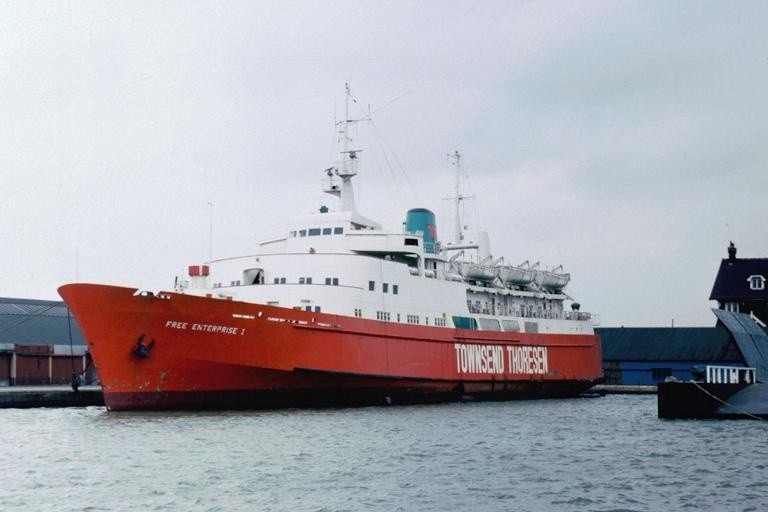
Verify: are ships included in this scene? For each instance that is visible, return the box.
[55,82,606,413]
